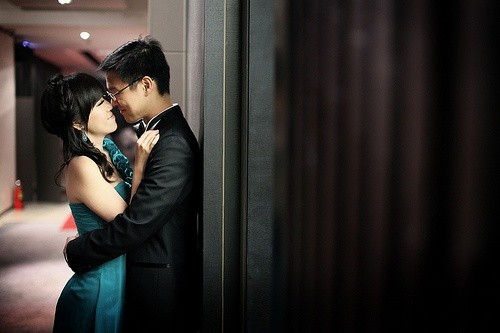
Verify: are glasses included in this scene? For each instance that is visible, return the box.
[105,77,154,102]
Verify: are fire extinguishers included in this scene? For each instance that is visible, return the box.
[13,180,24,210]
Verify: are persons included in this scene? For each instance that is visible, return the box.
[63,35,204,333]
[39,71,159,333]
